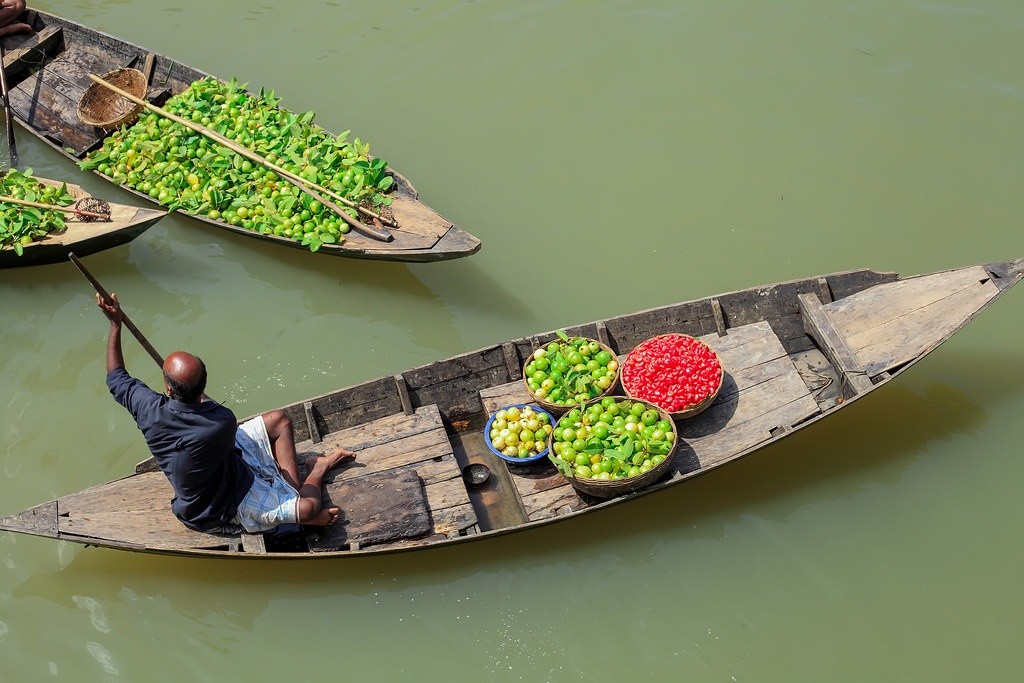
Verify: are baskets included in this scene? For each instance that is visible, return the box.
[484,404,557,465]
[548,395,678,496]
[76,68,148,130]
[620,332,724,420]
[523,337,621,416]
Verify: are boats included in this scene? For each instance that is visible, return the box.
[1,254,1022,561]
[0,167,171,267]
[0,1,483,264]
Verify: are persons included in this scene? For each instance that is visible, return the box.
[95,292,357,534]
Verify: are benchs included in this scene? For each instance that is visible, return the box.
[3,24,62,79]
[798,291,874,395]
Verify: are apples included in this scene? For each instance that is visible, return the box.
[97,83,364,238]
[553,398,674,480]
[525,340,618,406]
[490,406,552,458]
[11,185,57,245]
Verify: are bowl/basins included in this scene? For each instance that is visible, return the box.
[462,463,491,490]
[484,404,557,463]
